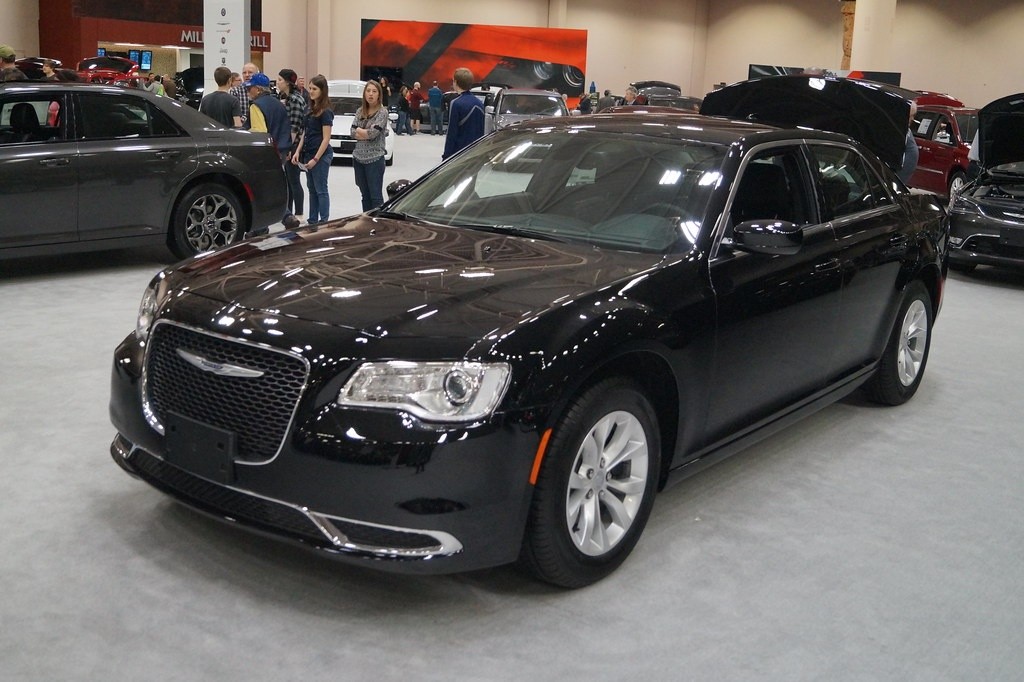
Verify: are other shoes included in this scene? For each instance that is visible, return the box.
[245,227,269,239]
[283,215,300,230]
[294,214,305,223]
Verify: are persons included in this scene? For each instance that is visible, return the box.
[350,79,388,213]
[577,92,593,114]
[803,66,823,74]
[291,74,334,225]
[967,128,980,178]
[595,89,615,114]
[619,86,639,106]
[395,81,424,136]
[0,44,29,81]
[40,60,59,80]
[428,80,447,135]
[379,76,391,108]
[897,99,919,185]
[562,94,568,101]
[199,63,310,239]
[442,67,486,161]
[131,71,177,98]
[481,83,490,91]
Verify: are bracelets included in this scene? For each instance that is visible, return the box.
[313,158,319,163]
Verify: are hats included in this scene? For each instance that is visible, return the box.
[0,46,16,59]
[280,69,297,87]
[245,74,270,86]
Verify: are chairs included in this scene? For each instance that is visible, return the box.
[733,162,798,227]
[502,97,521,113]
[7,103,42,142]
[820,172,850,211]
[100,112,166,135]
[545,175,631,226]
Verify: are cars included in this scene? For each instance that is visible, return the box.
[305,79,400,167]
[485,85,582,134]
[109,74,951,589]
[946,91,1024,285]
[1,82,301,262]
[899,90,982,202]
[574,80,704,114]
[404,86,505,124]
[0,56,137,89]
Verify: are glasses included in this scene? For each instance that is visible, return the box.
[233,80,242,82]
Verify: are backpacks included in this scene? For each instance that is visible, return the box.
[156,84,163,96]
